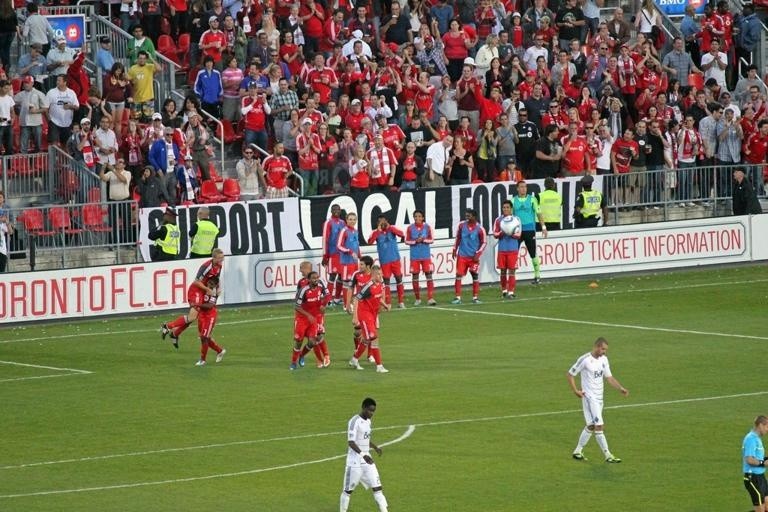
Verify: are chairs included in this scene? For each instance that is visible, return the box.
[236,121,248,137]
[81,203,112,233]
[688,73,705,90]
[217,119,240,143]
[156,34,200,87]
[0,79,61,178]
[200,179,224,202]
[87,188,101,202]
[50,204,80,236]
[198,162,222,181]
[21,207,53,238]
[132,186,141,204]
[221,176,241,201]
[57,169,81,197]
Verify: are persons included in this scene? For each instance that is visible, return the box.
[161,249,226,349]
[568,338,629,463]
[322,180,547,309]
[298,262,331,369]
[346,256,386,363]
[348,267,392,374]
[742,414,768,511]
[2,1,768,274]
[290,271,326,372]
[194,277,227,367]
[340,398,388,512]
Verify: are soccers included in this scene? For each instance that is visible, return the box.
[500,216,521,236]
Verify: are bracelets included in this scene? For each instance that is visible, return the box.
[360,450,368,459]
[760,459,765,467]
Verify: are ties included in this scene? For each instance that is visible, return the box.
[616,114,620,137]
[444,147,446,170]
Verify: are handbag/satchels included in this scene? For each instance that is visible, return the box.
[650,25,660,39]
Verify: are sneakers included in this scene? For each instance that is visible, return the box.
[349,360,364,369]
[334,300,343,306]
[290,363,296,370]
[367,356,375,362]
[509,295,516,299]
[414,300,422,305]
[451,298,462,304]
[678,202,687,208]
[317,361,323,368]
[502,293,508,298]
[195,360,206,366]
[472,299,481,303]
[605,455,622,462]
[531,278,541,285]
[216,349,226,362]
[160,323,169,340]
[323,358,330,367]
[427,298,437,305]
[572,452,588,460]
[686,201,696,207]
[299,354,304,367]
[168,331,179,348]
[399,304,405,308]
[376,366,389,373]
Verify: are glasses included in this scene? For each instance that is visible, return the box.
[407,104,415,106]
[585,126,594,130]
[270,54,279,58]
[1,118,13,125]
[549,105,559,109]
[599,47,608,50]
[165,133,174,136]
[717,110,723,115]
[244,151,253,155]
[519,114,528,117]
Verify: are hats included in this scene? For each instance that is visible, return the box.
[80,118,91,124]
[203,55,214,66]
[301,117,312,125]
[351,99,361,106]
[360,116,372,128]
[224,55,235,65]
[247,80,258,89]
[525,69,536,77]
[100,36,111,43]
[538,15,550,23]
[669,118,678,128]
[512,12,522,19]
[733,166,746,175]
[55,35,66,45]
[184,155,193,161]
[492,80,503,87]
[377,60,387,68]
[710,37,720,45]
[506,157,517,165]
[352,30,363,38]
[464,56,477,65]
[741,3,754,11]
[402,42,415,49]
[165,206,179,217]
[374,114,385,121]
[619,44,629,49]
[152,112,162,120]
[581,175,593,185]
[372,134,383,139]
[31,42,43,53]
[188,111,198,118]
[210,15,219,23]
[22,76,34,83]
[424,35,432,42]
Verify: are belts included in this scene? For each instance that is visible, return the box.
[401,179,415,182]
